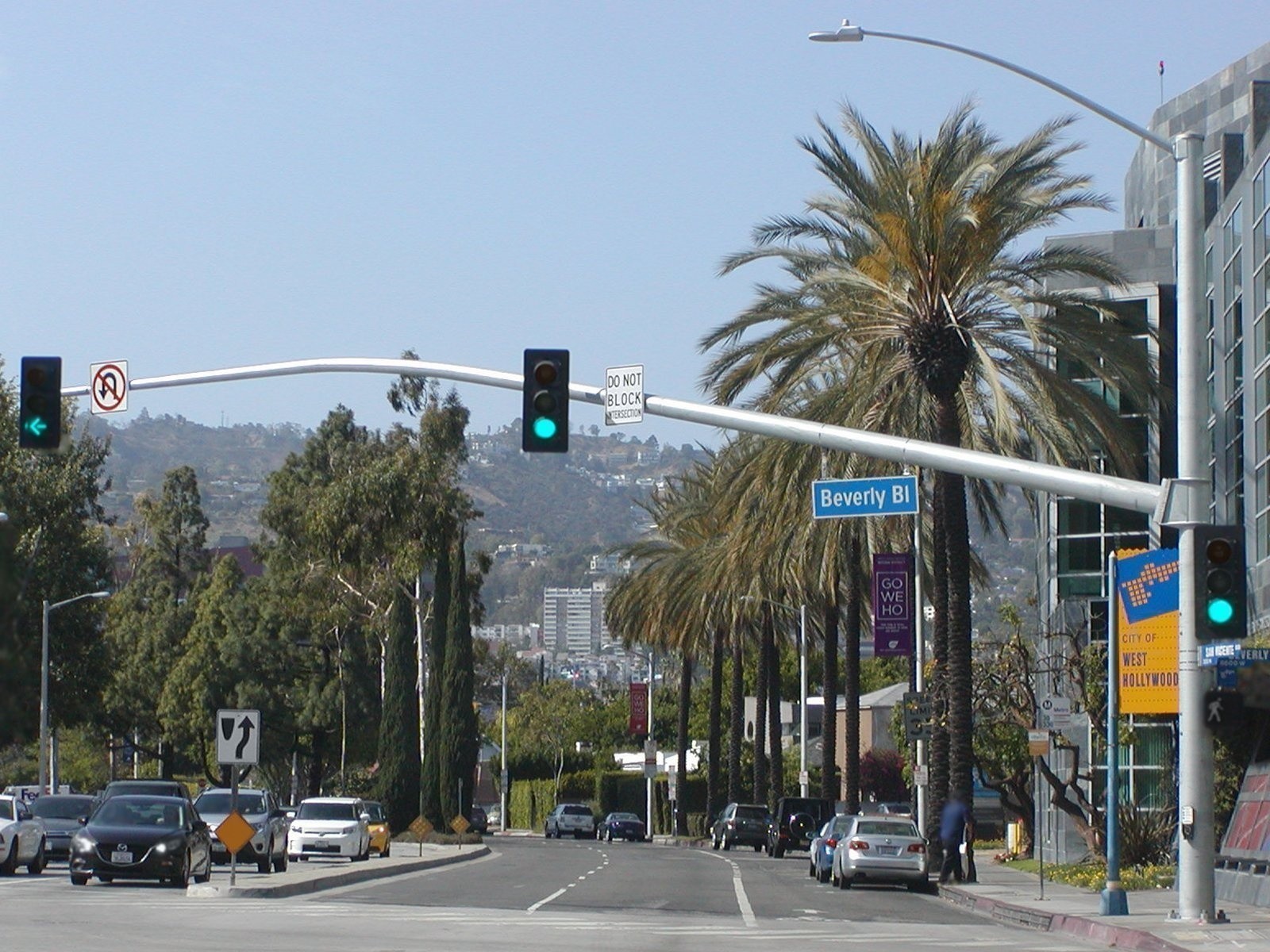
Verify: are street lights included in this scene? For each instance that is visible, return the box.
[38,590,112,799]
[739,595,812,794]
[809,21,1227,925]
[598,641,658,837]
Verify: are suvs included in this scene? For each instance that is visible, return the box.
[286,797,373,862]
[192,785,290,872]
[99,780,193,809]
[543,803,595,839]
[764,796,832,858]
[711,801,771,852]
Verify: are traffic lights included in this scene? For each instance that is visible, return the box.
[19,355,62,452]
[523,348,570,452]
[1194,524,1247,643]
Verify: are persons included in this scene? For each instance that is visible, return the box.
[938,789,977,886]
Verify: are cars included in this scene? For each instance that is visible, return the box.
[597,812,645,842]
[874,802,915,819]
[362,799,392,858]
[68,795,213,888]
[27,795,94,860]
[807,815,931,891]
[0,794,48,877]
[486,804,500,826]
[471,804,490,832]
